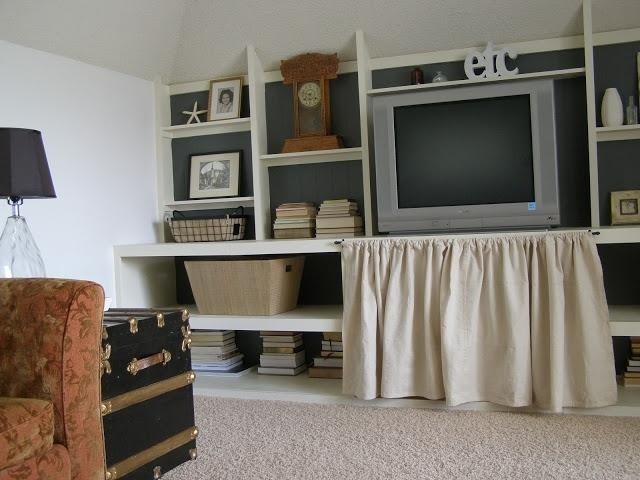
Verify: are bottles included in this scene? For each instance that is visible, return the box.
[410,68,424,85]
[432,71,447,83]
[601,88,624,127]
[626,95,637,125]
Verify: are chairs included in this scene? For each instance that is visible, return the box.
[0,279,106,478]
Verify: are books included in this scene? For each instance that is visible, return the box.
[191,330,342,379]
[617,336,640,387]
[272,198,364,238]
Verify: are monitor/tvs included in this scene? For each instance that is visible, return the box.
[371,77,561,236]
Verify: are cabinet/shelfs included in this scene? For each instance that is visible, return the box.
[148,34,369,239]
[586,29,640,228]
[116,236,640,416]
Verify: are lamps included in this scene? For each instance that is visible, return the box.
[0,127,57,277]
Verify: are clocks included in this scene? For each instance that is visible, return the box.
[279,51,340,153]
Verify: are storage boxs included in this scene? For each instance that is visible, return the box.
[101,307,198,480]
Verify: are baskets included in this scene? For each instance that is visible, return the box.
[166,206,250,243]
[183,255,306,316]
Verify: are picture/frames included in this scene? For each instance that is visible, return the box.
[187,149,242,200]
[206,77,243,121]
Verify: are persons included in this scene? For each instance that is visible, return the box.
[216,88,233,114]
